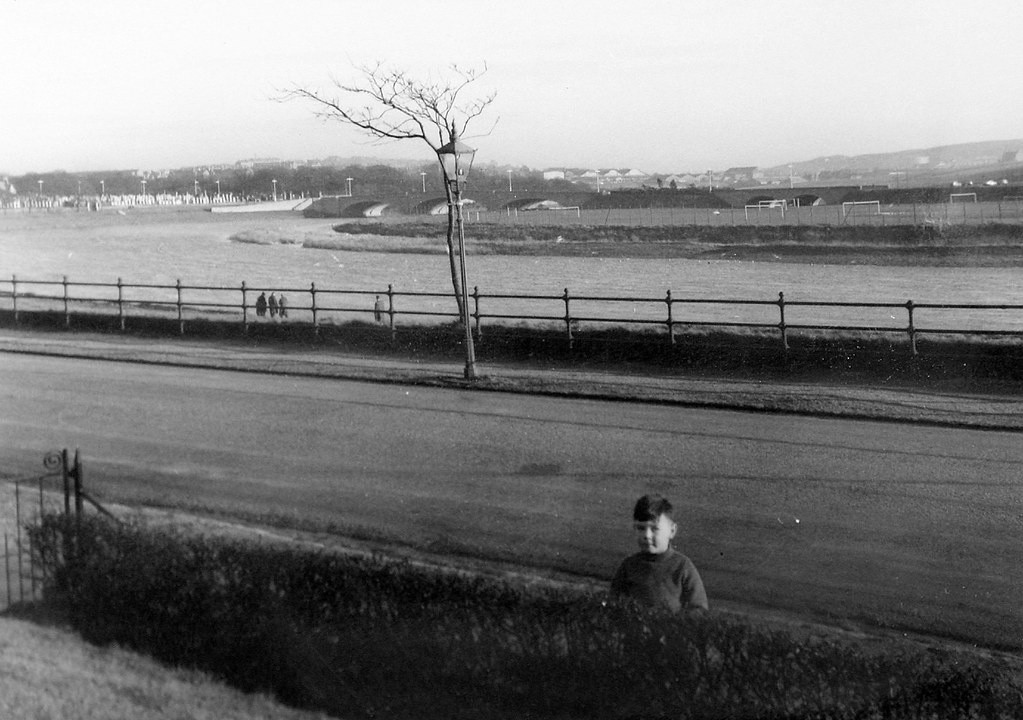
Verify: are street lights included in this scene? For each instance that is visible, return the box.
[33,165,517,194]
[435,120,482,379]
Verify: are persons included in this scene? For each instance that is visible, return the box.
[374,296,384,322]
[255,291,267,316]
[278,293,288,318]
[607,492,708,610]
[268,292,278,318]
